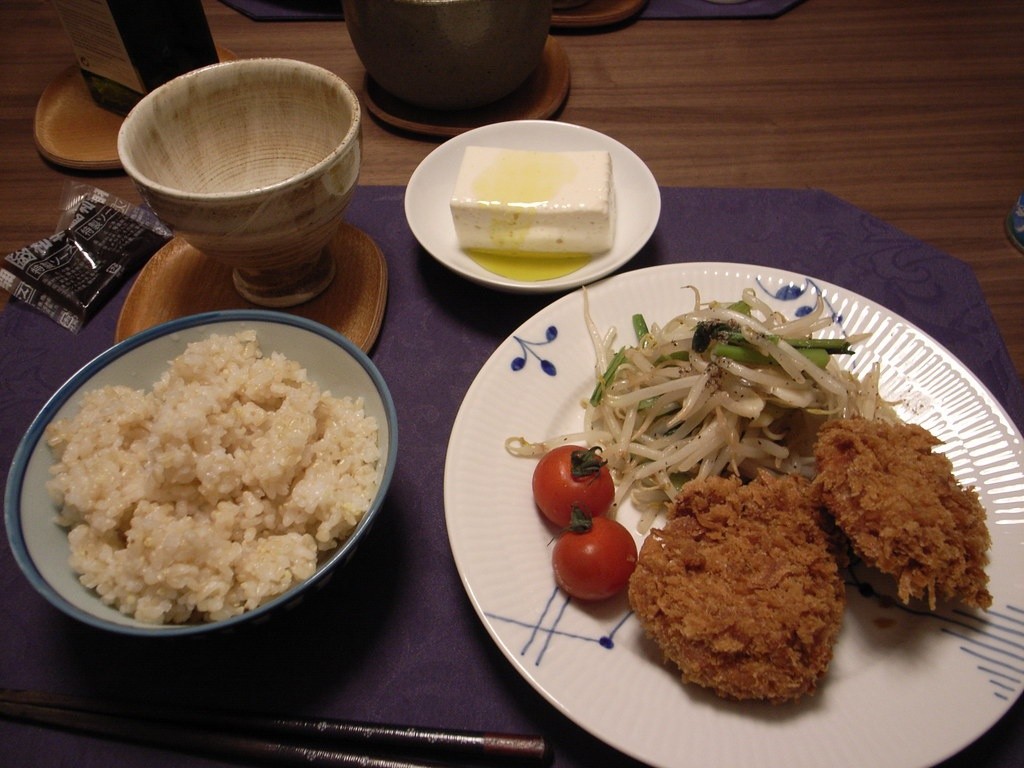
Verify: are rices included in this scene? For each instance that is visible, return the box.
[45,328,381,624]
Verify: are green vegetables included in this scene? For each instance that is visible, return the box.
[726,334,858,356]
[713,347,833,367]
[591,345,625,406]
[726,300,752,316]
[633,313,649,345]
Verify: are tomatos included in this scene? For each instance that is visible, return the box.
[532,445,638,601]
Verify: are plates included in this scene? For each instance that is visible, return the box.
[405,120,662,296]
[112,218,388,368]
[549,0,648,28]
[441,261,1024,768]
[364,32,578,139]
[31,40,242,170]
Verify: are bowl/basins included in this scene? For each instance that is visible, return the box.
[117,53,363,307]
[342,0,548,110]
[4,306,399,639]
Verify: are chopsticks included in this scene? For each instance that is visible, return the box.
[0,682,555,768]
